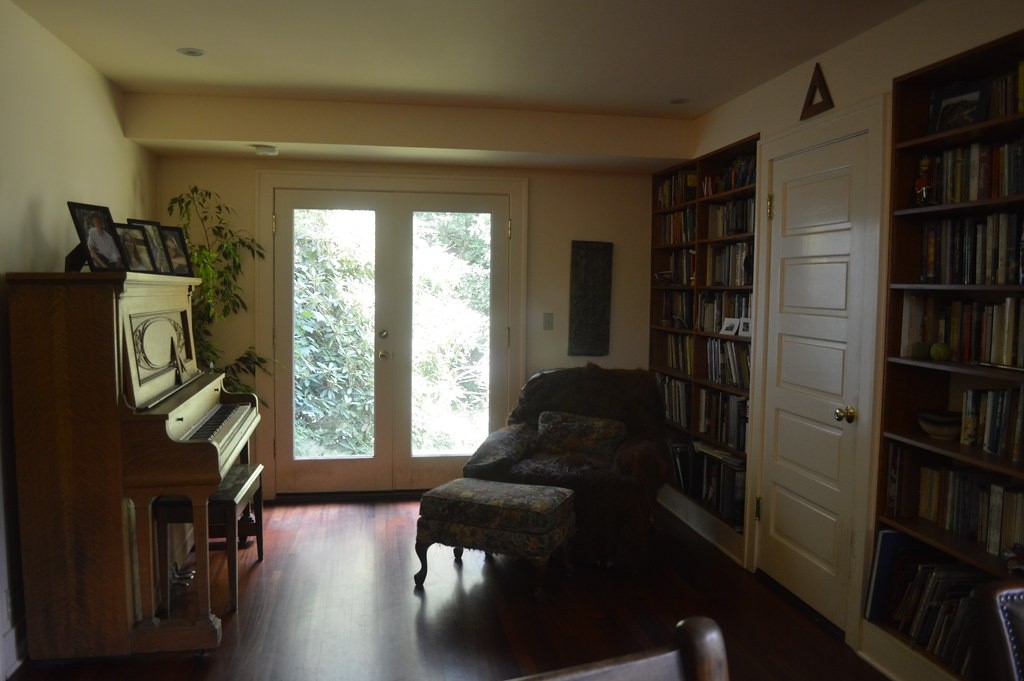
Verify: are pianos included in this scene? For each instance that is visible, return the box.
[1,269,264,663]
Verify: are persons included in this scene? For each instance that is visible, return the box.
[145,229,161,272]
[164,234,188,274]
[87,212,122,268]
[120,232,151,270]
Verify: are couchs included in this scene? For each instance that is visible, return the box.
[464,366,661,571]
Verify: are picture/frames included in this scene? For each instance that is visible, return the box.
[114,223,158,274]
[126,218,174,276]
[161,225,194,278]
[65,201,130,272]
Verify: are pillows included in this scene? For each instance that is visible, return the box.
[535,408,630,459]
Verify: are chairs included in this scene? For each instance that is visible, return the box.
[504,617,730,681]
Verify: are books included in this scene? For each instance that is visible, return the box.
[865,62,1024,681]
[655,155,756,533]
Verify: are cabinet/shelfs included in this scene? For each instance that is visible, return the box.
[647,134,761,567]
[861,29,1024,681]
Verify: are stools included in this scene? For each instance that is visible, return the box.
[152,462,266,613]
[414,478,578,601]
